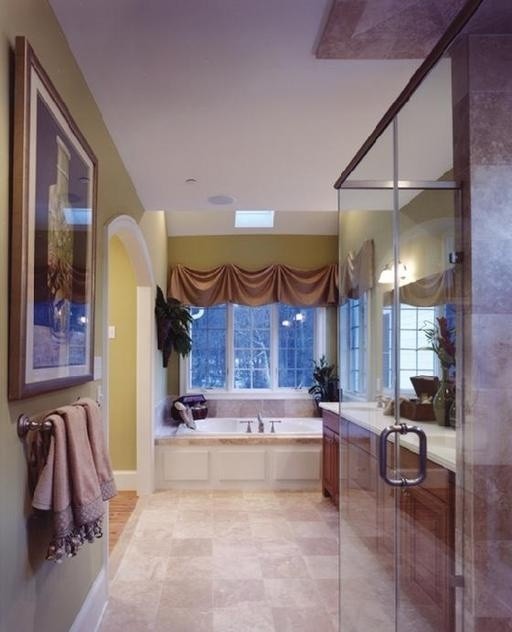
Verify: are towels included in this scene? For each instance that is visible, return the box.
[28,398,120,563]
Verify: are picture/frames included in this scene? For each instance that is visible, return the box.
[7,30,100,406]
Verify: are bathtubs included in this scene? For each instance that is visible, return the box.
[176,417,324,437]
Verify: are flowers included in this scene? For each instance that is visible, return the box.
[417,315,463,370]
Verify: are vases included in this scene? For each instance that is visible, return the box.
[431,367,457,426]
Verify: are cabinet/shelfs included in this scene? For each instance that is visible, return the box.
[338,420,377,547]
[379,440,455,631]
[318,410,347,515]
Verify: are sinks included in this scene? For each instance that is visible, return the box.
[417,433,457,449]
[341,406,377,412]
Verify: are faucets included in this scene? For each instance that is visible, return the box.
[377,396,391,407]
[257,414,264,433]
